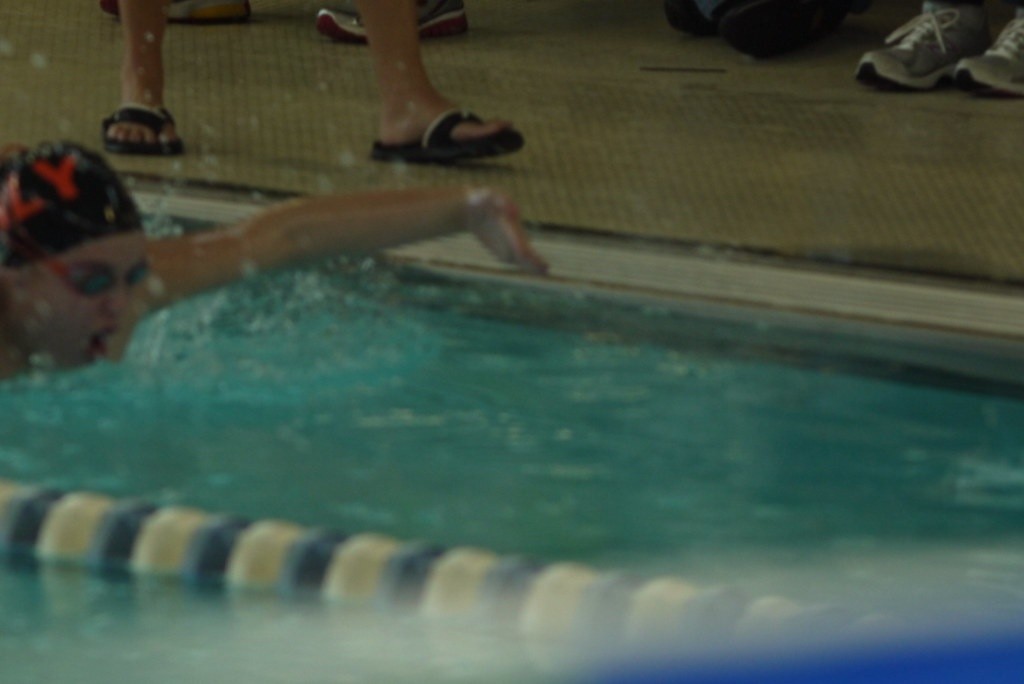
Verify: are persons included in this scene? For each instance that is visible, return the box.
[0,141,549,379]
[100,0,468,44]
[661,0,1024,99]
[101,0,524,161]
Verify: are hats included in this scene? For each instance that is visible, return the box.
[1,141,142,263]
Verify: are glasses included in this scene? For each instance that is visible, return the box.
[17,259,150,293]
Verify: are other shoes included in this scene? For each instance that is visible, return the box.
[318,9,369,47]
[663,1,719,42]
[714,1,854,56]
[419,8,469,38]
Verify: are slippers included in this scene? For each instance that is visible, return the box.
[368,104,522,163]
[102,104,186,159]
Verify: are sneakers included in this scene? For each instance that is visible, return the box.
[856,2,977,93]
[957,14,1024,103]
